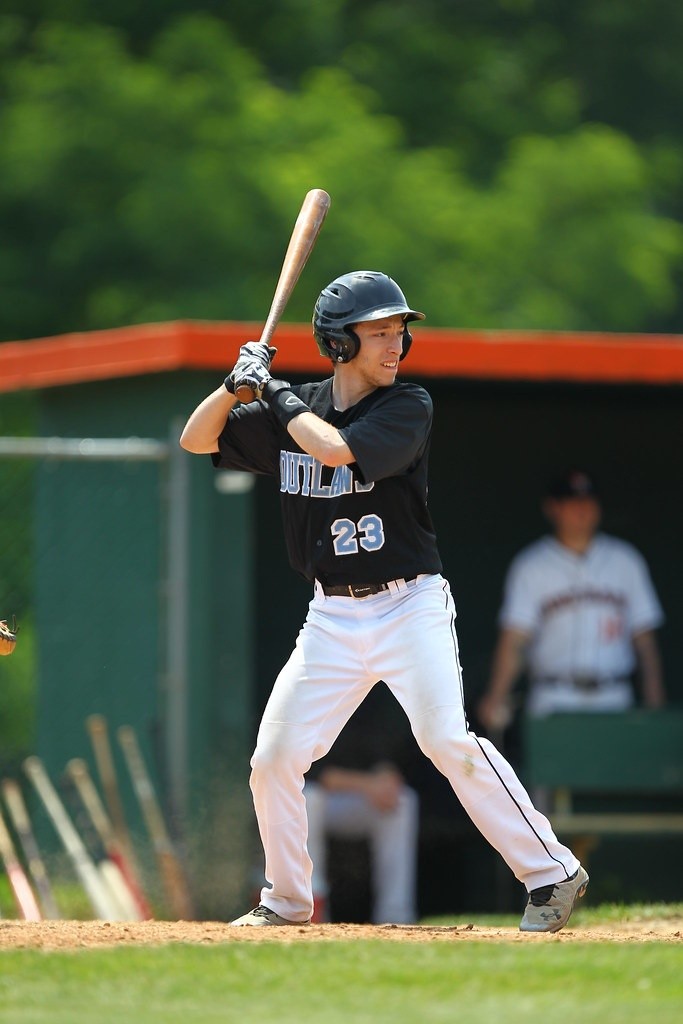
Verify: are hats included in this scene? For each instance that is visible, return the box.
[548,469,602,500]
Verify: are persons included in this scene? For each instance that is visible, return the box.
[276,471,662,926]
[181,271,589,933]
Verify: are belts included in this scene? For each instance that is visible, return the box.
[322,572,417,599]
[538,676,625,689]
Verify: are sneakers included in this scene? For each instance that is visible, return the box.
[519,865,589,933]
[230,907,312,927]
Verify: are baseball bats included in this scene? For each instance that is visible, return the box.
[235,187,331,405]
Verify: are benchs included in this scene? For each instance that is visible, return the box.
[531,714,681,838]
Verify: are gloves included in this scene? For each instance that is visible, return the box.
[223,340,277,395]
[251,362,312,431]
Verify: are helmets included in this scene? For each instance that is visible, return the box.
[312,272,426,364]
